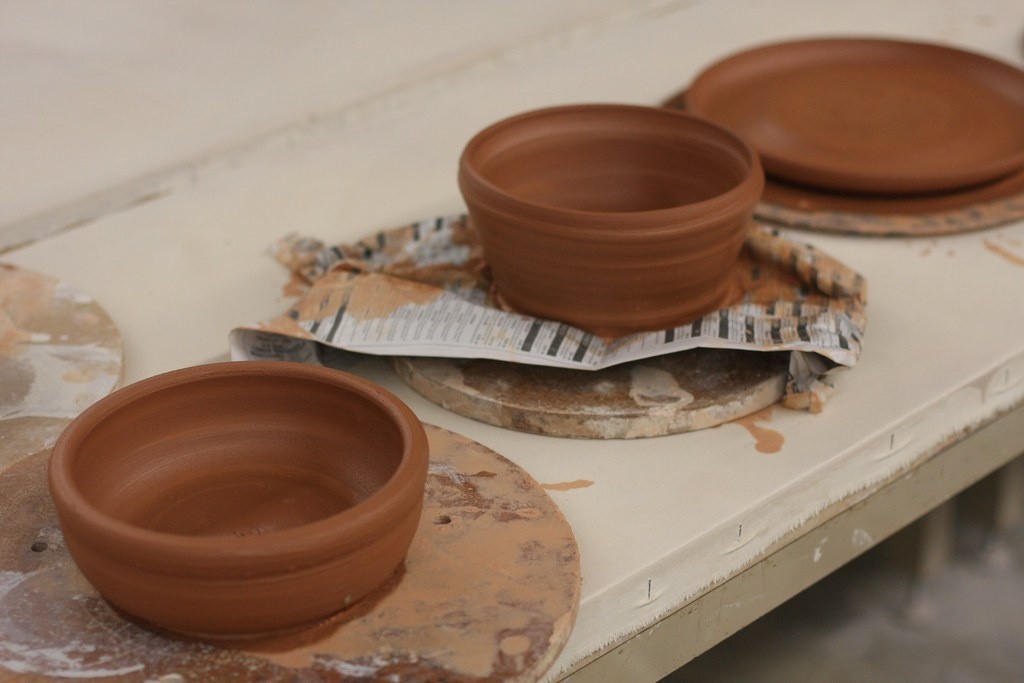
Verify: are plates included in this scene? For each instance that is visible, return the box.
[683,37,1024,199]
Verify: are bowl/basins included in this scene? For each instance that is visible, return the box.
[47,360,429,641]
[457,106,765,338]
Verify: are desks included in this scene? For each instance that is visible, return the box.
[0,0,1024,683]
